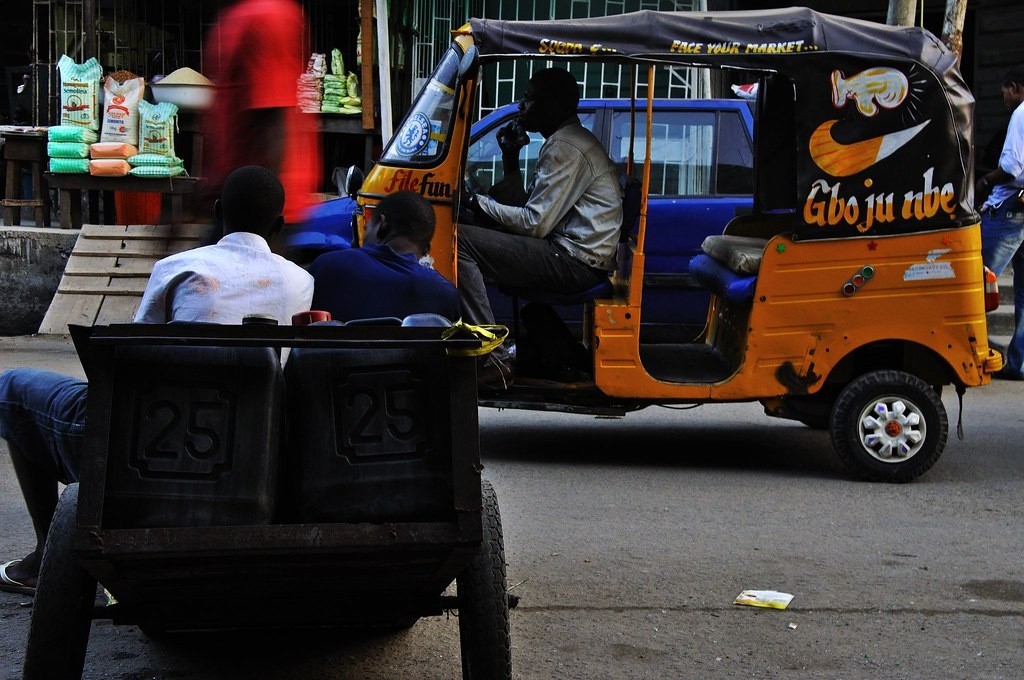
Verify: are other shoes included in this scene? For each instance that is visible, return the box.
[478,360,515,393]
[991,366,1024,380]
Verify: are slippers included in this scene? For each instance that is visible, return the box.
[0,558,38,596]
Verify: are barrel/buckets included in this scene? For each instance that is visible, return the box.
[284,311,441,523]
[114,191,161,225]
[104,315,283,526]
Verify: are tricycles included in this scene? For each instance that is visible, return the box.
[23,313,523,680]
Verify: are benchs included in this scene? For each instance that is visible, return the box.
[687,234,771,299]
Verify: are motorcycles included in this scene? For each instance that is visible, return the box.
[346,7,1005,484]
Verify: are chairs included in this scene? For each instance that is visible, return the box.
[499,178,644,297]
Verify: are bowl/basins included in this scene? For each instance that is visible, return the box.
[151,83,215,109]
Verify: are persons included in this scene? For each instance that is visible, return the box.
[0,166,315,597]
[975,65,1024,380]
[309,191,459,323]
[459,68,623,388]
[204,1,311,175]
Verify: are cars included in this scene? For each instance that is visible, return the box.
[301,96,758,321]
